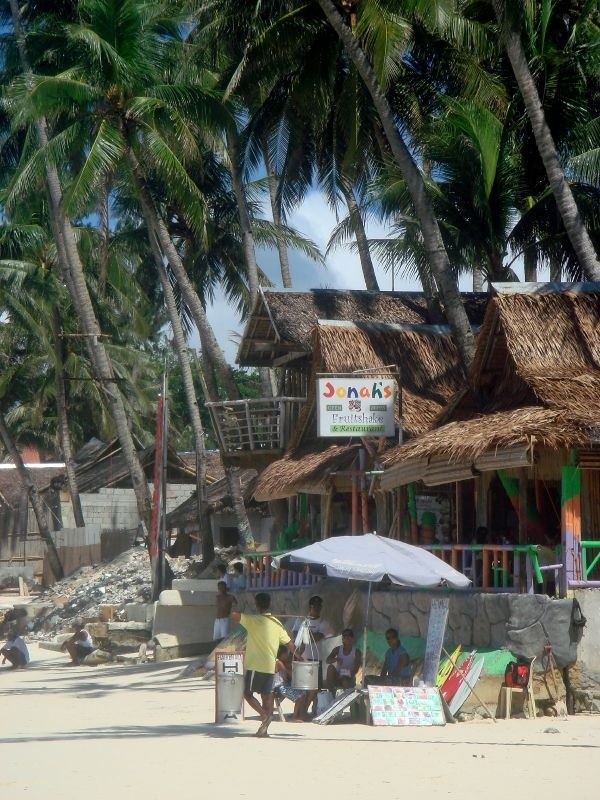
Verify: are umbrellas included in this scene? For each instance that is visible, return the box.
[270,531,472,691]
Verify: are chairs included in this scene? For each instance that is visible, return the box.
[500,655,537,720]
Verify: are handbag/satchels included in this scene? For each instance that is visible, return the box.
[505,659,528,695]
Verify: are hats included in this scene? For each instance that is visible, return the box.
[342,629,354,637]
[310,596,322,605]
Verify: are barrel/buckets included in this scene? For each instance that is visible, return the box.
[292,626,320,691]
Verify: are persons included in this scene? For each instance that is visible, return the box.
[0,630,29,670]
[182,521,203,559]
[359,628,412,687]
[61,617,94,667]
[418,524,559,596]
[214,562,362,738]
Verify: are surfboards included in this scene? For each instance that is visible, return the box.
[438,645,484,716]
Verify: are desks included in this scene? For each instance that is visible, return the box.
[358,688,372,724]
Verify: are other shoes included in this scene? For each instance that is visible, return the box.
[256,715,273,737]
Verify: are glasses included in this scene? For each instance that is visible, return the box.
[386,636,395,640]
[311,605,321,609]
